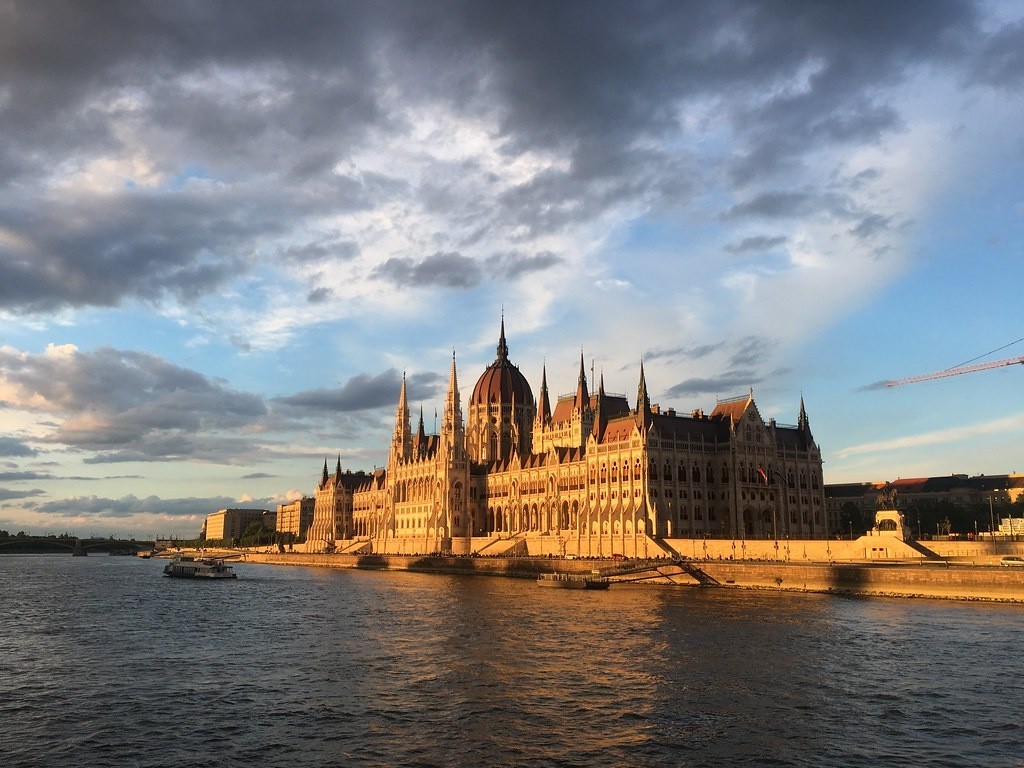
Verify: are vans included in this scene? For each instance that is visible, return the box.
[194,554,203,561]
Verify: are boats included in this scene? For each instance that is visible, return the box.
[162,556,237,579]
[536,572,611,589]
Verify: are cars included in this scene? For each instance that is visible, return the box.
[1000,556,1024,567]
[610,554,624,559]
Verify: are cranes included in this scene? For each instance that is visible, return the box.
[885,338,1024,388]
